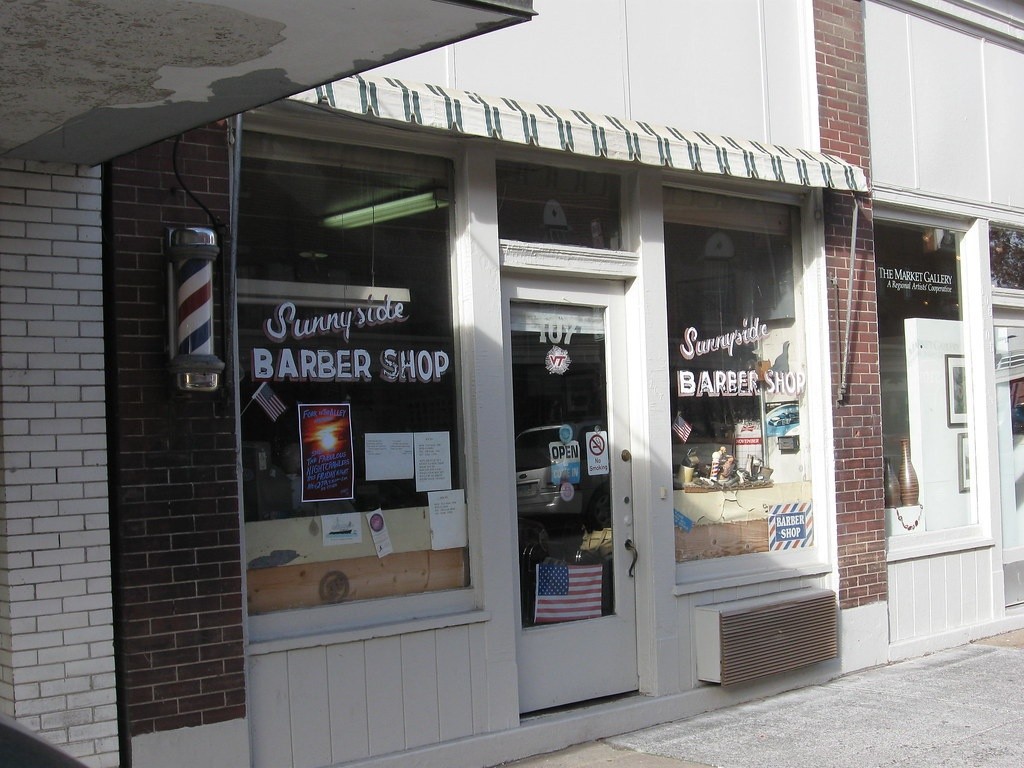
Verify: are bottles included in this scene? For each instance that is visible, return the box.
[884,456,900,508]
[898,439,919,506]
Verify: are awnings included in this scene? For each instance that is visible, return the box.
[289,76,871,194]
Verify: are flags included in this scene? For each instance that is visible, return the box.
[533,563,603,622]
[672,417,692,443]
[251,382,286,422]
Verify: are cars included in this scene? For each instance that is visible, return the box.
[766,402,802,456]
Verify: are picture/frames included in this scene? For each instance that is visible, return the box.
[958,433,971,494]
[946,354,968,429]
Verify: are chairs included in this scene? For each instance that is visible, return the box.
[242,441,306,520]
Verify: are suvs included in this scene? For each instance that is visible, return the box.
[514,420,614,535]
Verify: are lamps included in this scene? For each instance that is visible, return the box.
[160,226,224,393]
[317,187,450,232]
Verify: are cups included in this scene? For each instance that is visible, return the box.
[677,465,694,484]
